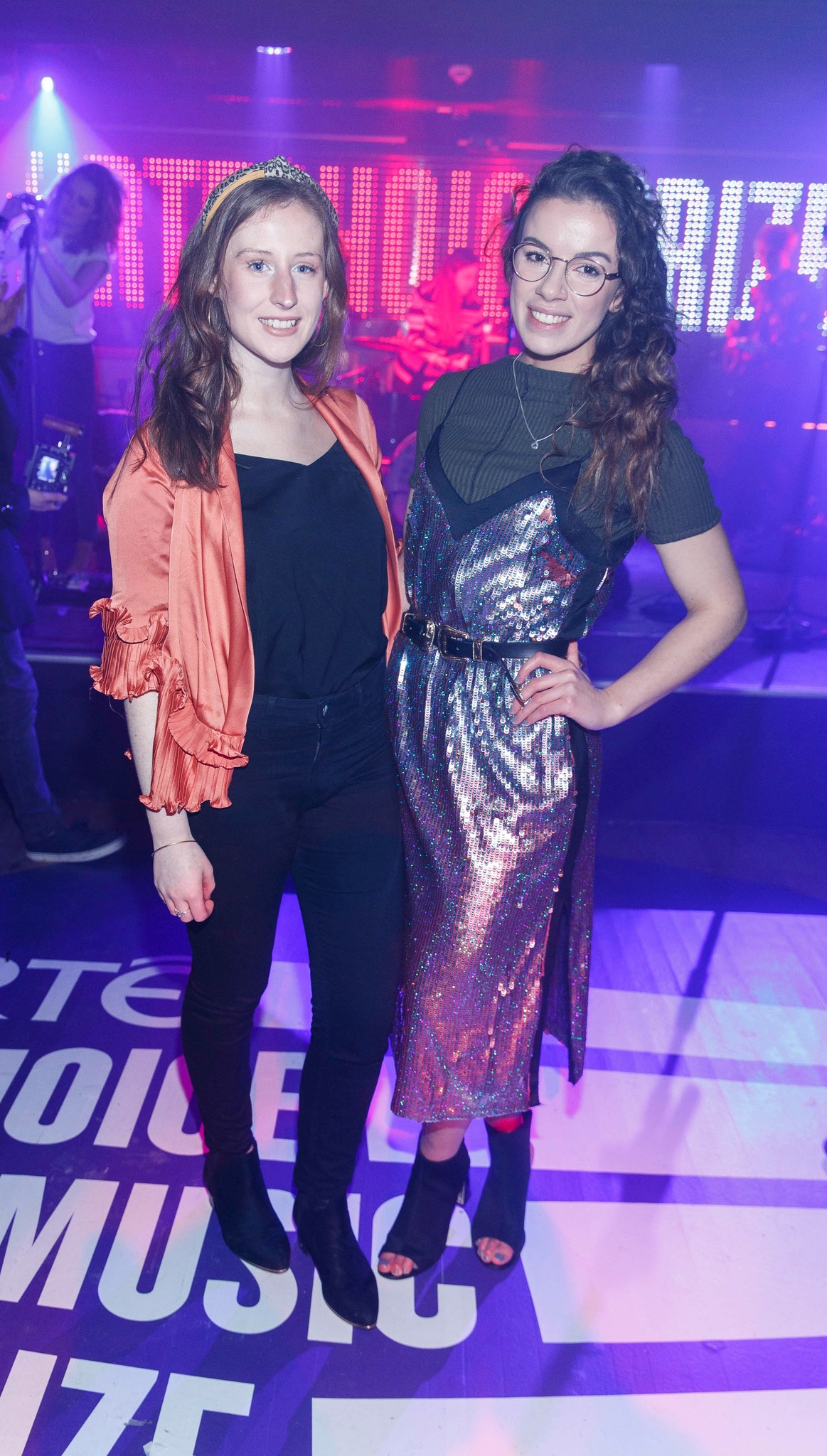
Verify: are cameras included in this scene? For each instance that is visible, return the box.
[26,445,76,494]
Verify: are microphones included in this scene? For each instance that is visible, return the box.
[20,193,49,209]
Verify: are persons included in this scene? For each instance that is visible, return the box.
[0,161,123,868]
[724,227,817,567]
[389,250,489,431]
[379,149,750,1280]
[91,165,406,1330]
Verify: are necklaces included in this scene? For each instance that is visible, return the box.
[512,352,590,448]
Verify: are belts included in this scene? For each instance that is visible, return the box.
[400,613,566,662]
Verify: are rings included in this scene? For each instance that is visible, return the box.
[174,908,190,917]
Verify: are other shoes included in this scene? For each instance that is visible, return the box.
[24,820,126,863]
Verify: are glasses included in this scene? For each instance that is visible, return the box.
[511,243,623,296]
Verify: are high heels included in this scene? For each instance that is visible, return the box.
[293,1191,378,1328]
[204,1138,292,1274]
[466,1109,532,1268]
[378,1137,472,1281]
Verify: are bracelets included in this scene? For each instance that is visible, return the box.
[38,247,49,256]
[151,840,196,856]
[0,215,9,230]
